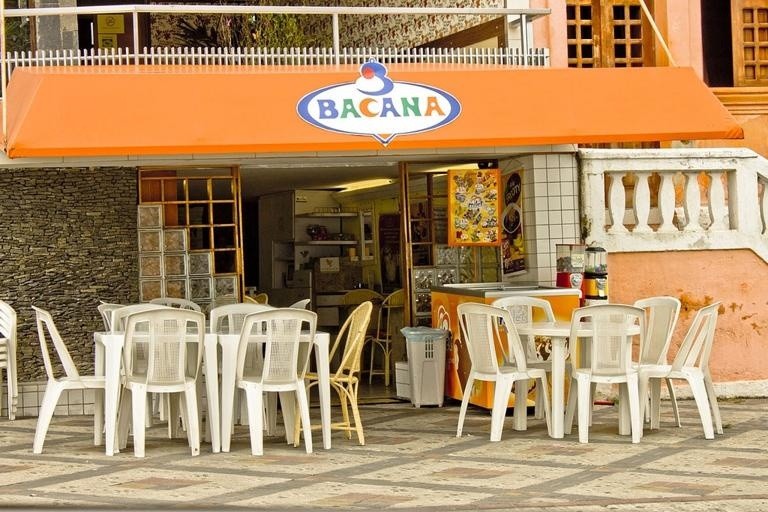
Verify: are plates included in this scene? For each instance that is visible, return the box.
[500,202,522,234]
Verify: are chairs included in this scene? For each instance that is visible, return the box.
[210,303,273,333]
[0,300,20,422]
[149,297,202,315]
[228,306,315,455]
[242,293,269,304]
[289,298,311,309]
[26,304,122,455]
[292,301,375,447]
[456,302,551,443]
[99,303,125,331]
[113,310,207,455]
[490,295,568,421]
[617,296,682,429]
[343,288,404,386]
[567,304,647,445]
[639,301,724,440]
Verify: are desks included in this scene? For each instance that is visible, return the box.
[505,320,643,439]
[92,328,333,457]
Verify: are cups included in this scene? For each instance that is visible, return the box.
[347,247,355,257]
[308,224,344,240]
[353,282,365,288]
[365,248,369,256]
[313,207,359,213]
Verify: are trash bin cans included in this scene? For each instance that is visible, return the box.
[399,326,449,408]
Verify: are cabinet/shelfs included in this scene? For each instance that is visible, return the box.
[256,188,361,325]
